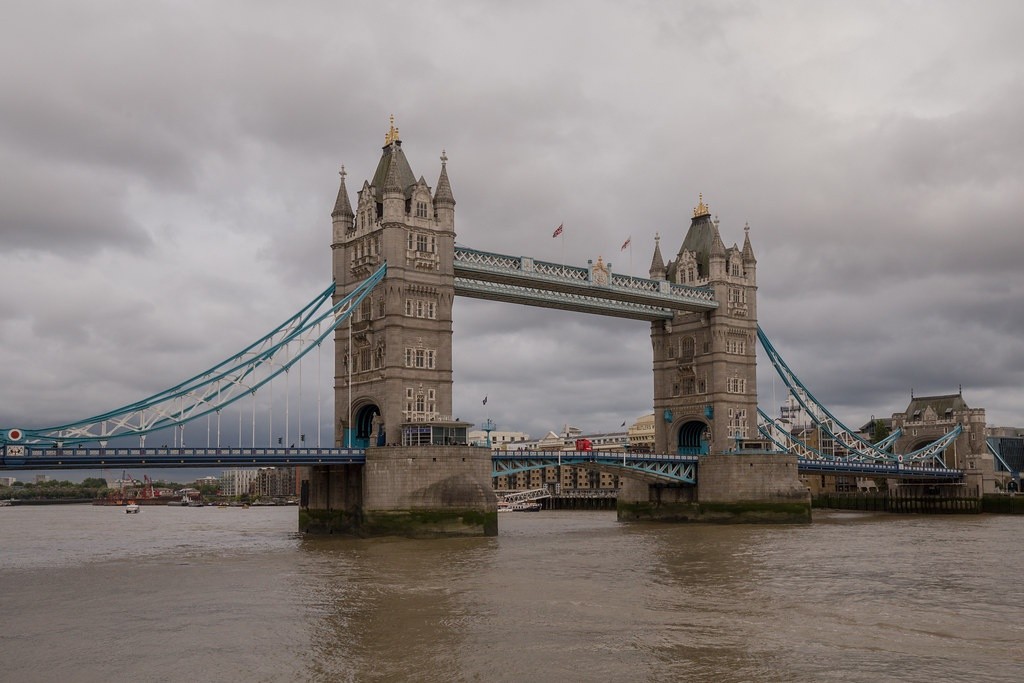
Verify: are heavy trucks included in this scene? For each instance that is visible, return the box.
[575,438,593,451]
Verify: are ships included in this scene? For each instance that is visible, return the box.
[92,470,173,505]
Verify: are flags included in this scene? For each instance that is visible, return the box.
[621,422,625,427]
[621,236,631,252]
[482,395,487,405]
[553,223,563,238]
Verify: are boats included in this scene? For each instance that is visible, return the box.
[125,502,140,514]
[166,496,298,509]
[498,498,542,513]
[0,499,21,507]
